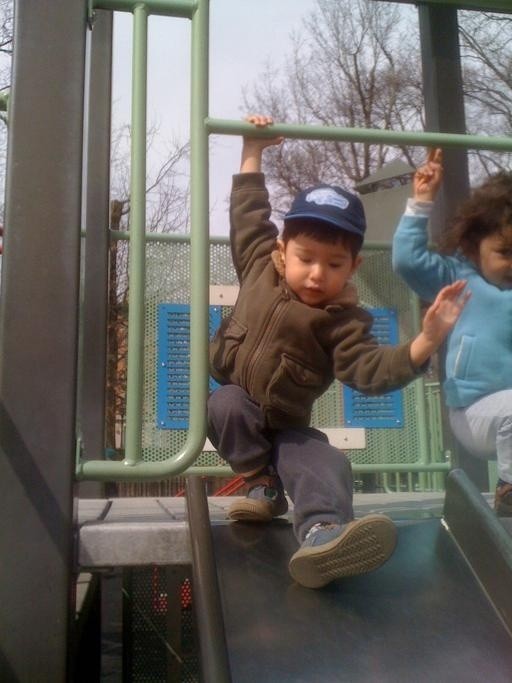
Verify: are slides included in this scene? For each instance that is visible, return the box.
[185,466,510,681]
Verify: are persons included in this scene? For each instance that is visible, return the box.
[204,113,474,592]
[388,146,511,518]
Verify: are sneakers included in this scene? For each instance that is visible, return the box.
[230,484,290,521]
[288,514,399,591]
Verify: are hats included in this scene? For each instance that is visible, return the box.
[280,183,367,239]
